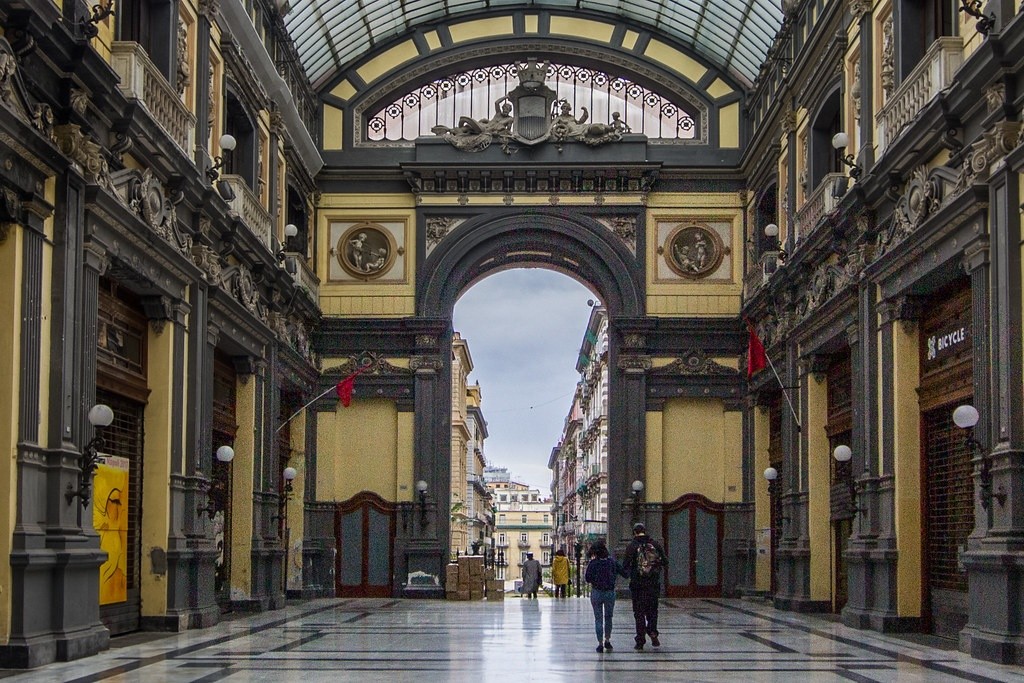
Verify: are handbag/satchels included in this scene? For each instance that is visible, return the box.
[537,572,542,585]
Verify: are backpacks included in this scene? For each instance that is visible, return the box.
[636,541,661,577]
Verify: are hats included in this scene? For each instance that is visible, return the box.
[526,552,533,557]
[632,523,646,536]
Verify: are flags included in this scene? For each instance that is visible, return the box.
[746,318,767,376]
[339,364,368,409]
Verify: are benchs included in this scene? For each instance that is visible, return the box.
[402,587,443,598]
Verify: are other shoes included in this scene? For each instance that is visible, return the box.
[605,641,613,649]
[596,645,604,653]
[650,631,660,646]
[634,642,644,650]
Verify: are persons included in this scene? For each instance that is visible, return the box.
[364,248,387,273]
[349,232,367,270]
[678,245,698,272]
[586,540,627,655]
[521,551,543,599]
[550,549,569,600]
[609,111,631,140]
[693,231,708,268]
[489,91,513,130]
[544,102,576,139]
[624,522,663,652]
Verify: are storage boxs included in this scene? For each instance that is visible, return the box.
[445,555,505,601]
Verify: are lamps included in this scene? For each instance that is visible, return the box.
[417,480,430,532]
[764,225,788,262]
[64,403,113,510]
[205,135,237,184]
[263,468,296,508]
[834,444,867,516]
[832,133,862,182]
[197,445,234,519]
[764,467,791,524]
[952,404,1007,508]
[633,481,644,517]
[276,224,298,264]
